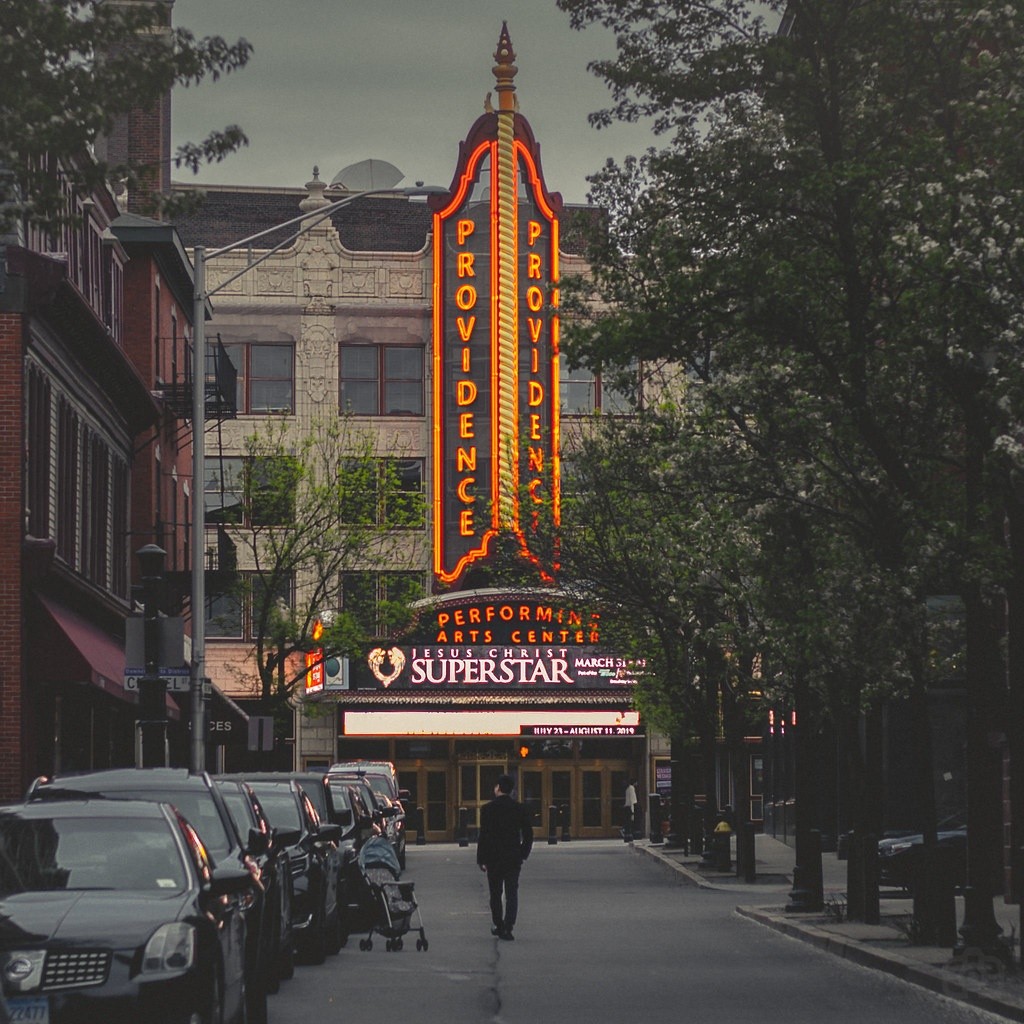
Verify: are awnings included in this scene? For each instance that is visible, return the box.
[25,584,182,724]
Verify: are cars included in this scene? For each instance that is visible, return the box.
[2,798,258,1024]
[878,819,969,895]
[29,758,411,979]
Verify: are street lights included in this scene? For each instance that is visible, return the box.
[192,178,450,782]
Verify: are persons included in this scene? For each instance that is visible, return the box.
[623,778,638,843]
[476,775,534,941]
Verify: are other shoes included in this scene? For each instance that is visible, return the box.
[491,925,514,941]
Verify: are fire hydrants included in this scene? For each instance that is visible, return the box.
[714,821,734,873]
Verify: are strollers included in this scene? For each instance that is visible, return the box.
[347,832,431,955]
